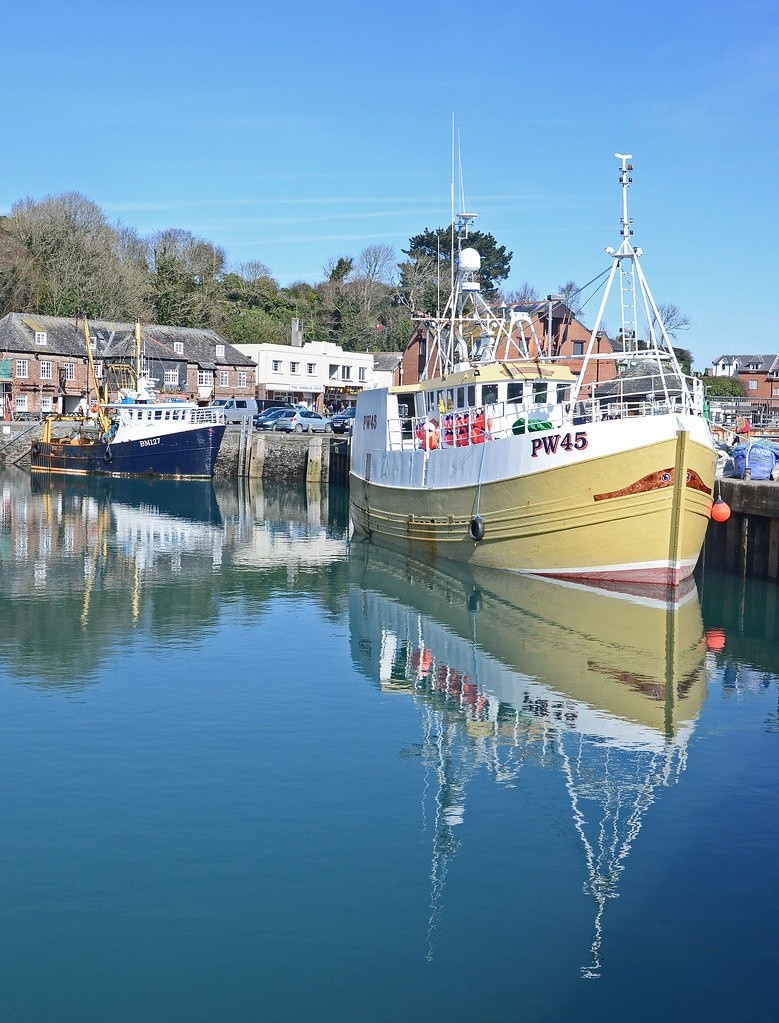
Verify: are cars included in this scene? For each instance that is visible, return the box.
[277,411,333,433]
[254,406,285,427]
[254,408,294,432]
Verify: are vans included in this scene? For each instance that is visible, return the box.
[331,407,356,434]
[207,398,258,424]
[285,403,308,411]
[257,399,295,414]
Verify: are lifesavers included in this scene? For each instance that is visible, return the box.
[33,442,41,453]
[468,519,485,542]
[104,451,112,461]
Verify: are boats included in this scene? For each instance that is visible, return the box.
[347,113,722,586]
[29,471,226,633]
[347,537,712,983]
[29,309,230,479]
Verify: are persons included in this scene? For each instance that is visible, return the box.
[732,412,750,446]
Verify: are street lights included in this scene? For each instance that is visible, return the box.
[595,334,602,391]
[547,294,565,363]
[620,329,633,369]
[728,363,731,377]
